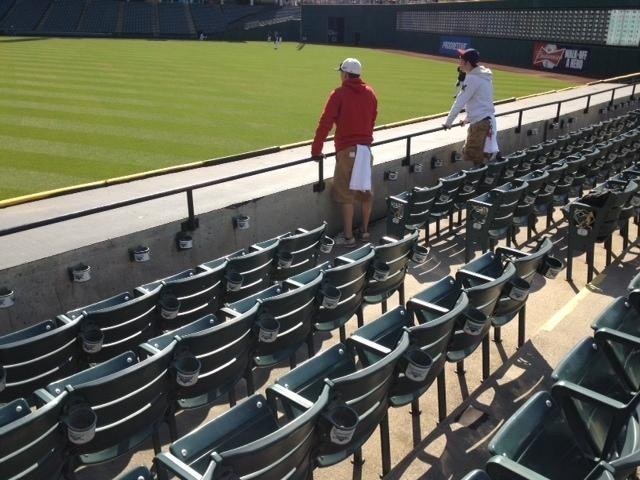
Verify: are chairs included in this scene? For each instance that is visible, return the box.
[386,112,640,283]
[2,1,301,36]
[0,220,640,479]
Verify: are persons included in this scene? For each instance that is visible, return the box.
[453,64,465,98]
[309,55,380,249]
[267,29,272,42]
[440,49,496,169]
[273,30,280,49]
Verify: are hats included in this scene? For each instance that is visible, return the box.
[456,48,481,65]
[334,57,362,75]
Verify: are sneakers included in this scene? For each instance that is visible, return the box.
[332,232,358,248]
[352,224,371,242]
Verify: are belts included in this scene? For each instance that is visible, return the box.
[483,116,491,120]
